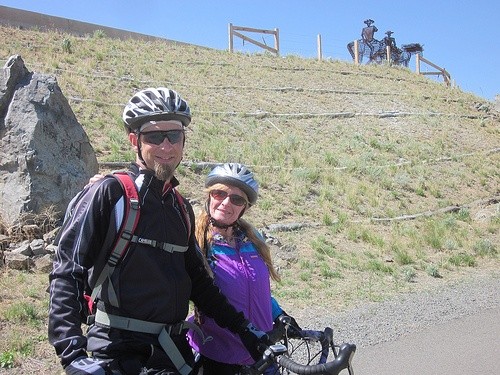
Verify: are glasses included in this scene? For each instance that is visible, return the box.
[210,189,247,206]
[139,129,183,146]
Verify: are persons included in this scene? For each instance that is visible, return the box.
[361,19,378,60]
[186,162,302,375]
[48,87,272,375]
[379,31,395,58]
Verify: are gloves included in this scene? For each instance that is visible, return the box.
[64,355,107,375]
[237,319,275,363]
[279,315,302,340]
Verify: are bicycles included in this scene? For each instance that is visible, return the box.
[241,342,357,375]
[266,314,337,365]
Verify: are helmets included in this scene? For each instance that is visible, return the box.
[204,162,258,207]
[122,87,191,129]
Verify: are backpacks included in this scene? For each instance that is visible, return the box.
[45,170,193,327]
